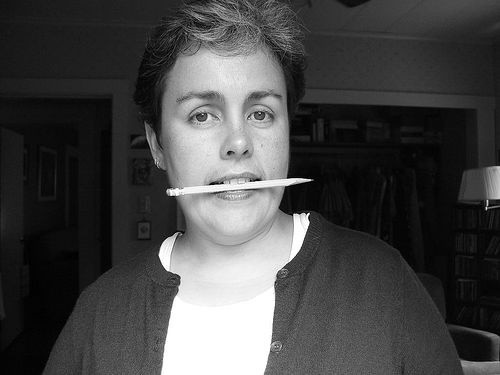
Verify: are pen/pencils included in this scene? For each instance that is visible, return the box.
[165,177,314,196]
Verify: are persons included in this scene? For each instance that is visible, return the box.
[42,0,464,375]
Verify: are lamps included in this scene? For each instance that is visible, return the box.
[457,166,500,211]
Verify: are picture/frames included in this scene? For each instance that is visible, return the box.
[36,145,58,203]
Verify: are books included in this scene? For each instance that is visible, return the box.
[452,231,478,324]
[478,295,500,333]
[291,113,445,147]
[454,203,500,230]
[484,235,500,257]
